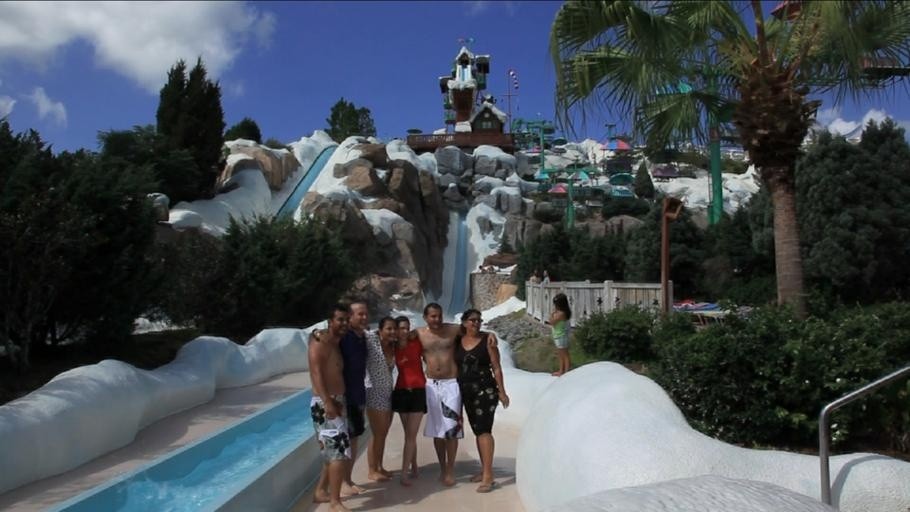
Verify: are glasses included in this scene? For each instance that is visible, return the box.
[469,319,483,323]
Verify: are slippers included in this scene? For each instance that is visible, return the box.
[476,482,498,492]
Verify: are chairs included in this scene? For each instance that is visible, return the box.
[673,299,760,325]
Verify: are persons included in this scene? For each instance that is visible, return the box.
[454,308,510,494]
[479,265,496,274]
[392,316,427,486]
[312,299,371,496]
[361,315,417,481]
[408,302,498,486]
[529,269,542,283]
[544,292,572,376]
[307,303,355,512]
[540,269,551,284]
